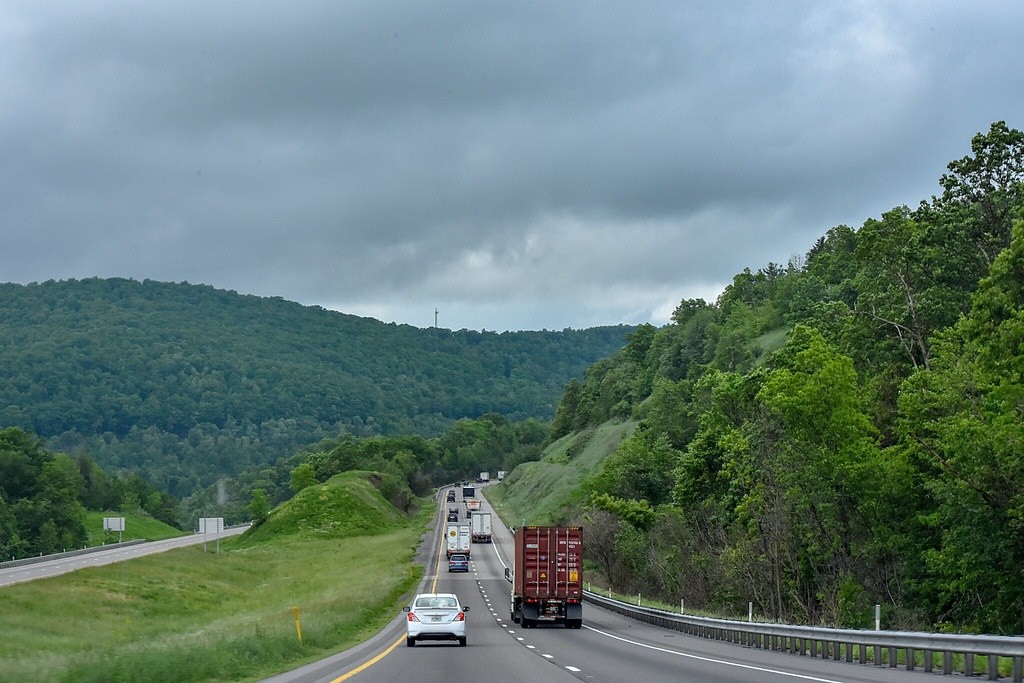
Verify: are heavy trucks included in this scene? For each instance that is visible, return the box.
[504,525,584,629]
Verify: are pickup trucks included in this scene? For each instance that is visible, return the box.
[455,480,470,487]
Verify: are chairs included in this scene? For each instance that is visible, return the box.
[420,600,430,607]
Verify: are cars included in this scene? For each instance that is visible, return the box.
[447,513,458,522]
[446,490,456,502]
[448,554,469,572]
[402,592,470,647]
[449,506,458,514]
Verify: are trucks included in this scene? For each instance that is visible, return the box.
[463,488,474,498]
[463,499,482,518]
[480,472,489,483]
[469,511,492,543]
[444,521,472,559]
[498,471,509,481]
